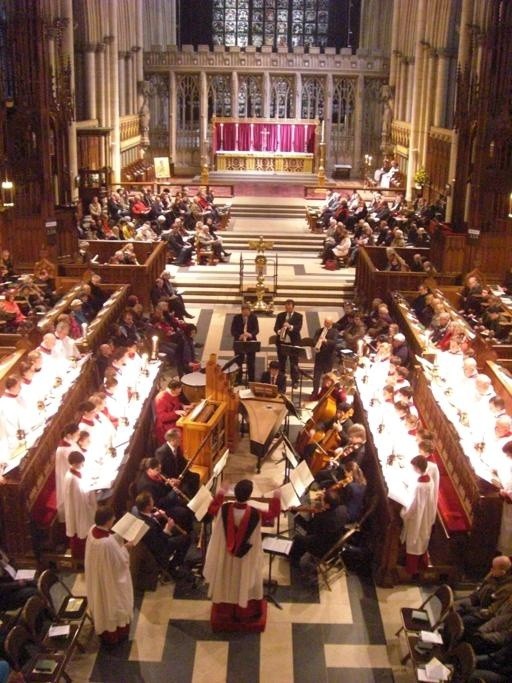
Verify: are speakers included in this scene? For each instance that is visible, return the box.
[170,162,175,179]
[331,164,352,180]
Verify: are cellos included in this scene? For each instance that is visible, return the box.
[295,380,342,459]
[308,407,355,479]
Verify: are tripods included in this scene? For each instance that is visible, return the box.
[269,416,301,538]
[295,373,312,411]
[264,552,284,613]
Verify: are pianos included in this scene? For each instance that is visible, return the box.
[176,398,229,478]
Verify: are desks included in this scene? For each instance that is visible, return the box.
[334,163,351,180]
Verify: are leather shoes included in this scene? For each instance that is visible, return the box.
[190,248,232,264]
[186,313,204,348]
[293,382,318,399]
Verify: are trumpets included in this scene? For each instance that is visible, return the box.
[314,327,329,353]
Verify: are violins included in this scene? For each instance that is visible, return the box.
[281,502,329,514]
[330,477,353,490]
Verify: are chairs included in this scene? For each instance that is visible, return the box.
[394,582,476,682]
[309,488,380,593]
[194,240,214,265]
[334,246,353,267]
[3,567,93,682]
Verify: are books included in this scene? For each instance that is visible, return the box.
[411,610,428,623]
[413,630,443,655]
[417,657,451,682]
[15,569,36,581]
[36,660,55,671]
[50,624,70,639]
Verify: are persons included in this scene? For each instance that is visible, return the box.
[75,188,231,264]
[204,479,264,620]
[315,189,448,263]
[84,506,133,646]
[1,552,37,605]
[0,243,512,574]
[378,158,391,185]
[380,160,400,188]
[452,555,512,682]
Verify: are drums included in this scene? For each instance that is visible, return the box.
[180,373,207,404]
[216,358,239,386]
